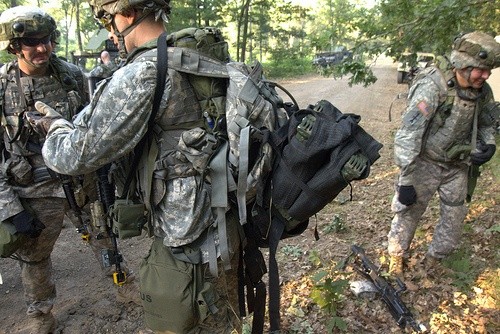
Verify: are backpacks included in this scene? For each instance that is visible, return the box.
[133,49,384,249]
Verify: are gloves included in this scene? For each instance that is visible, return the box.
[471,144,496,166]
[11,213,45,237]
[25,101,64,138]
[398,185,417,205]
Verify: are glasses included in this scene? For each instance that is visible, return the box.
[18,35,52,46]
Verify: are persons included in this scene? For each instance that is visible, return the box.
[26,0,246,334]
[0,6,134,334]
[386,30,500,283]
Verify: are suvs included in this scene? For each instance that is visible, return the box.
[397,53,435,84]
[313,44,353,67]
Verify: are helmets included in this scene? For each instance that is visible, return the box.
[0,5,56,49]
[450,31,500,69]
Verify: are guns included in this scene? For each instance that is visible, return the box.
[59,173,85,234]
[351,245,421,334]
[87,76,126,287]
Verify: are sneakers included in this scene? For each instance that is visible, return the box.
[424,256,454,280]
[28,312,56,334]
[388,257,405,282]
[120,263,135,283]
[113,281,142,306]
